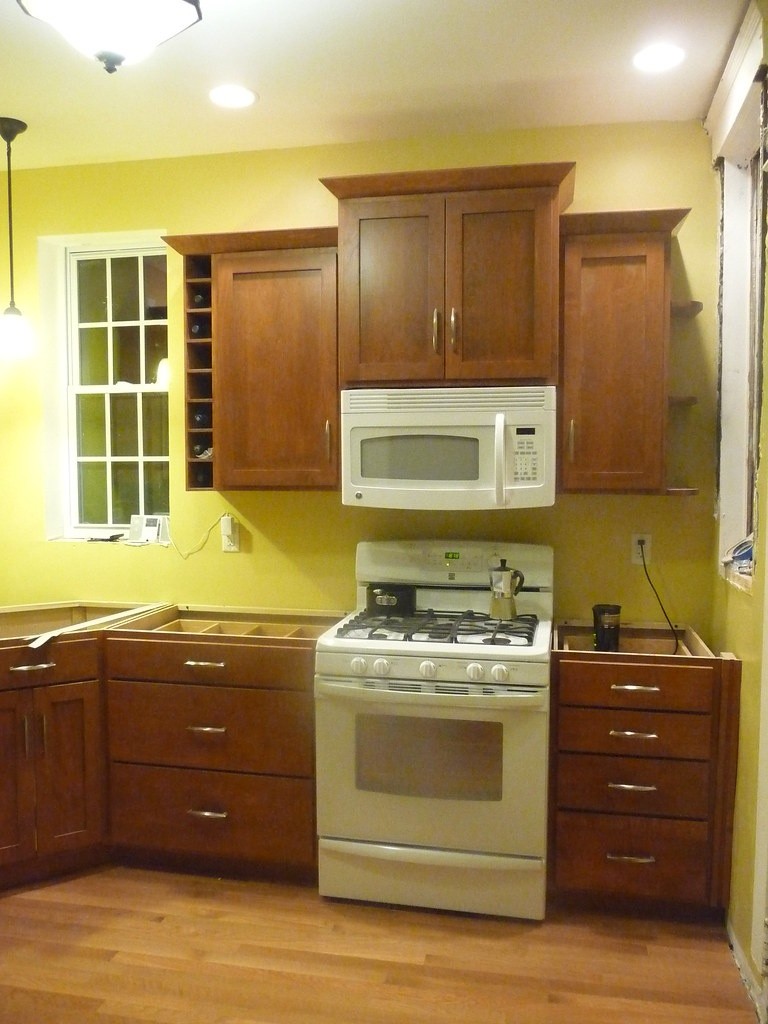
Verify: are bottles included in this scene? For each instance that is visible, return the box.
[192,324,209,336]
[193,409,208,424]
[194,444,210,455]
[194,294,204,305]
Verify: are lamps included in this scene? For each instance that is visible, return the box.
[0,118,40,356]
[15,0,203,73]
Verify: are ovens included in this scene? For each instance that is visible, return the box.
[311,648,553,923]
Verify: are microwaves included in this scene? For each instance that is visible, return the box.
[341,386,557,512]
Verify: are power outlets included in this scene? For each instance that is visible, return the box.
[222,523,240,552]
[630,532,653,566]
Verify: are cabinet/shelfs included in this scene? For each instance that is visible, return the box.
[157,161,704,497]
[551,615,743,922]
[1,604,359,893]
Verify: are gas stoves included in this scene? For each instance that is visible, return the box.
[314,539,554,659]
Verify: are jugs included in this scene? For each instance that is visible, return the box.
[488,559,525,621]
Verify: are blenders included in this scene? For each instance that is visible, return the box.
[592,604,620,651]
